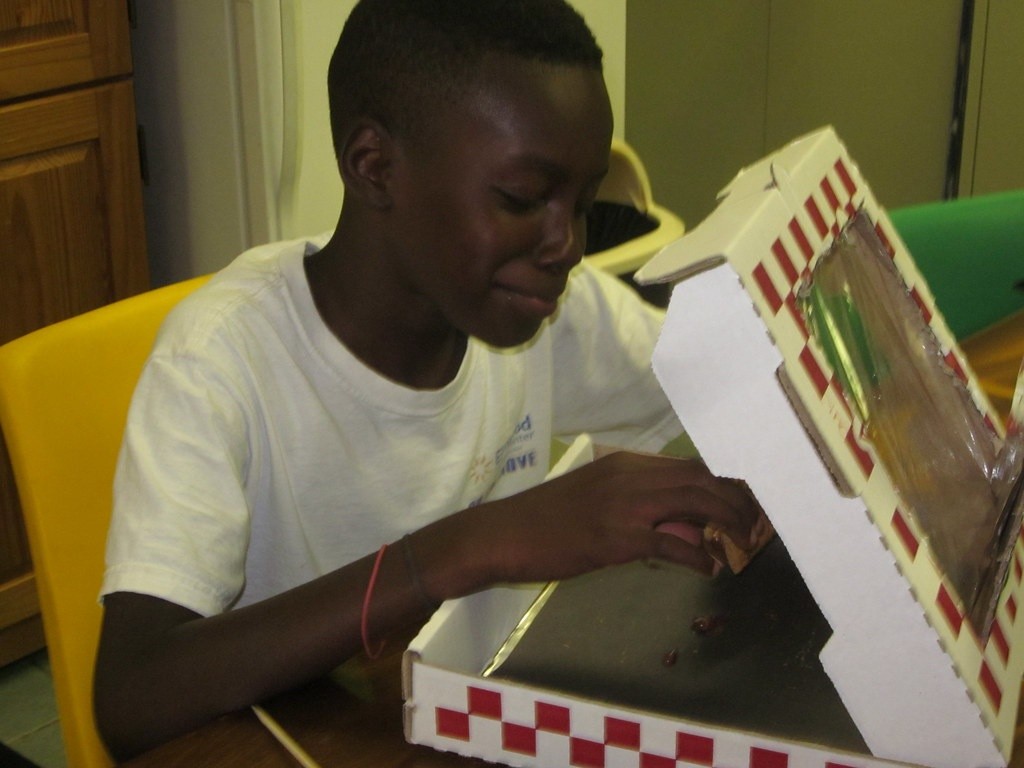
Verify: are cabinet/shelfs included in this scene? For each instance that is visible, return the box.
[0,0,150,668]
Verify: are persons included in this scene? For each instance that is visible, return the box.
[93,0,764,768]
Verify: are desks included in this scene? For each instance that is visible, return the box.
[117,298,1024,768]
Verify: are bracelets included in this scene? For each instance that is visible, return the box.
[401,530,435,611]
[360,543,386,660]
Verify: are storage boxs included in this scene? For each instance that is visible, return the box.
[401,125,1024,768]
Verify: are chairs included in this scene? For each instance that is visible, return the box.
[886,189,1024,342]
[0,270,223,768]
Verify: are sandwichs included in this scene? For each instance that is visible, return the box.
[701,481,777,575]
